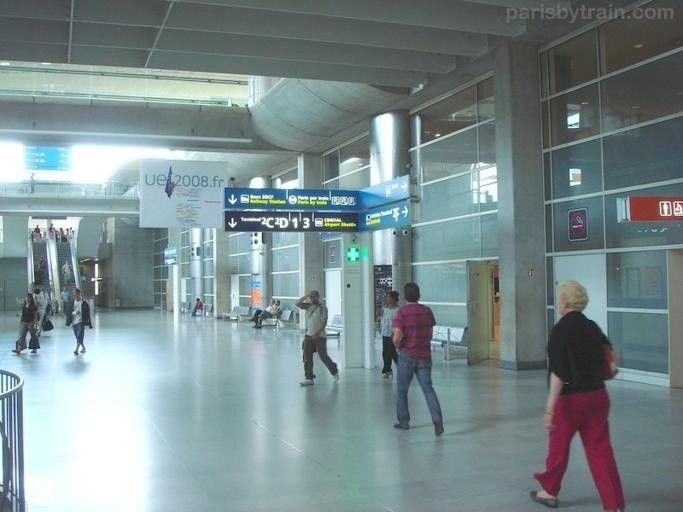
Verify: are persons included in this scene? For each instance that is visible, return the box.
[530,282,625,511]
[252,300,280,328]
[30,172,35,193]
[249,299,276,322]
[295,290,339,385]
[11,257,92,355]
[380,291,398,377]
[394,283,444,435]
[32,223,74,244]
[192,298,204,315]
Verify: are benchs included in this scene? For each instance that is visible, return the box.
[200,304,212,316]
[180,302,191,313]
[221,306,251,322]
[263,310,294,330]
[430,326,467,361]
[325,314,343,339]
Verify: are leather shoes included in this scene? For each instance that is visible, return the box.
[530,490,558,508]
[394,424,409,430]
[435,426,444,436]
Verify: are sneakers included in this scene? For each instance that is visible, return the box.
[300,379,314,386]
[332,374,339,383]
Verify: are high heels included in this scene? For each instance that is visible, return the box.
[382,371,394,379]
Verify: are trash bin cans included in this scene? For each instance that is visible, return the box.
[83,297,95,321]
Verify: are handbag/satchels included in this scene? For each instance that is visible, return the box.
[15,337,28,351]
[43,319,54,331]
[599,343,619,381]
[28,336,41,349]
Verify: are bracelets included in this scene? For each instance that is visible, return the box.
[545,412,552,417]
